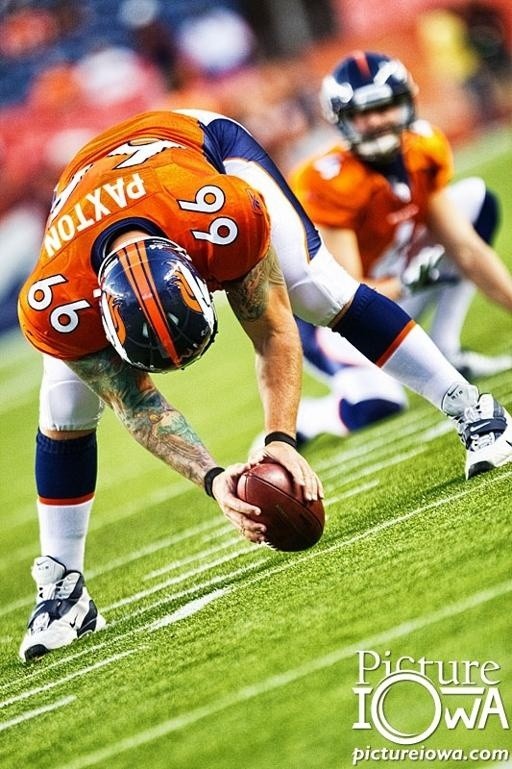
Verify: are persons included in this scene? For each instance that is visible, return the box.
[3,0,512,335]
[249,50,512,465]
[19,109,512,666]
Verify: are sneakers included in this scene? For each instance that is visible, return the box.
[248,397,321,460]
[18,555,108,665]
[450,351,512,382]
[440,376,512,480]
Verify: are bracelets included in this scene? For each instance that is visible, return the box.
[205,466,226,500]
[264,431,299,453]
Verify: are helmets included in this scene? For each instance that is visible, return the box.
[317,51,420,160]
[98,234,221,373]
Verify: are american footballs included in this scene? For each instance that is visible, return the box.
[238,463,325,550]
